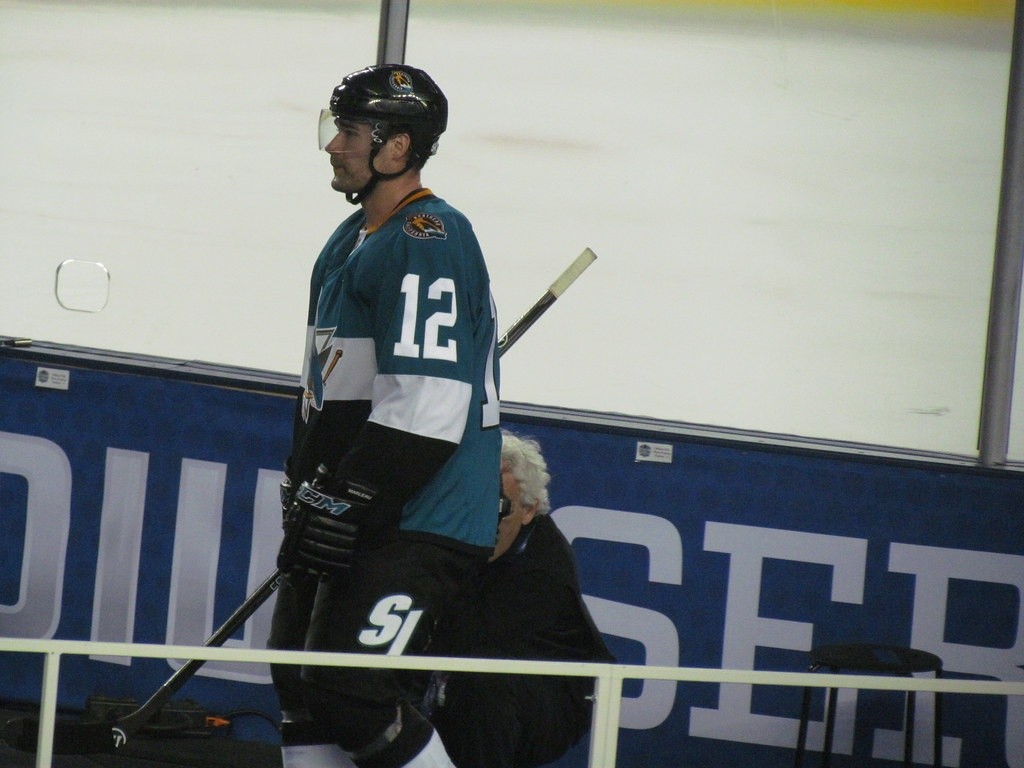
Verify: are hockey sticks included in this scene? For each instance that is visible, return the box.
[0,247,596,756]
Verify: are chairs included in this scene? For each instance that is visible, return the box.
[794,642,946,768]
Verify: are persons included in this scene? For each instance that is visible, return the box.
[266,66,503,768]
[433,428,620,768]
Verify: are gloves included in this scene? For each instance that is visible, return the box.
[276,464,377,583]
[279,479,296,535]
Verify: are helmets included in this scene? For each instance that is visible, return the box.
[329,64,448,156]
